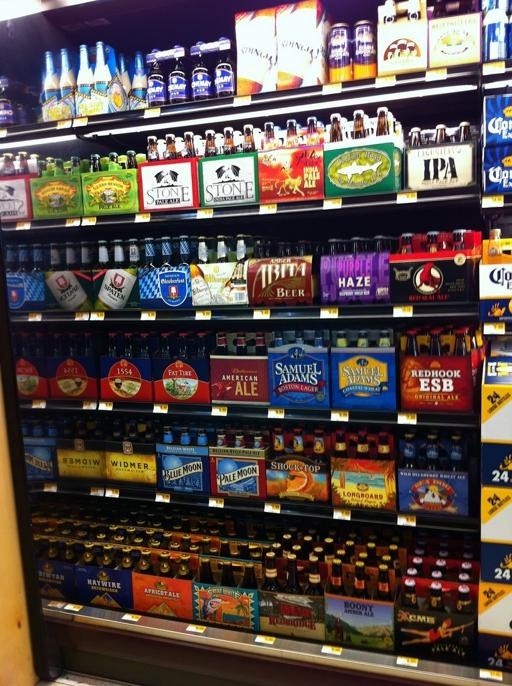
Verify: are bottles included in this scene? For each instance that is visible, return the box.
[0,34,237,130]
[23,412,469,472]
[20,326,480,359]
[325,0,512,85]
[0,108,475,179]
[6,230,481,270]
[33,505,473,616]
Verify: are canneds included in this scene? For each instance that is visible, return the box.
[327,22,352,83]
[352,19,377,81]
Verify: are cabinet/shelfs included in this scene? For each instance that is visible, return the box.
[0,0,512,686]
